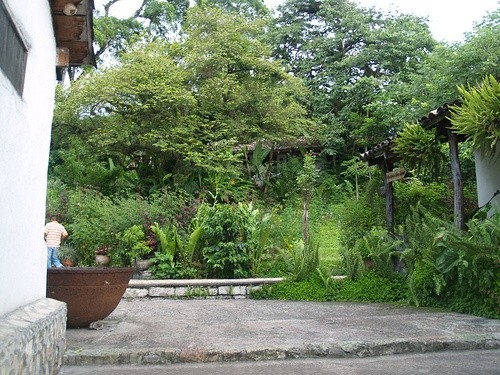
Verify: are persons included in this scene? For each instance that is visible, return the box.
[44,215,69,270]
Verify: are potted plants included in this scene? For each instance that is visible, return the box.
[59,242,80,265]
[94,245,111,266]
[132,242,154,271]
